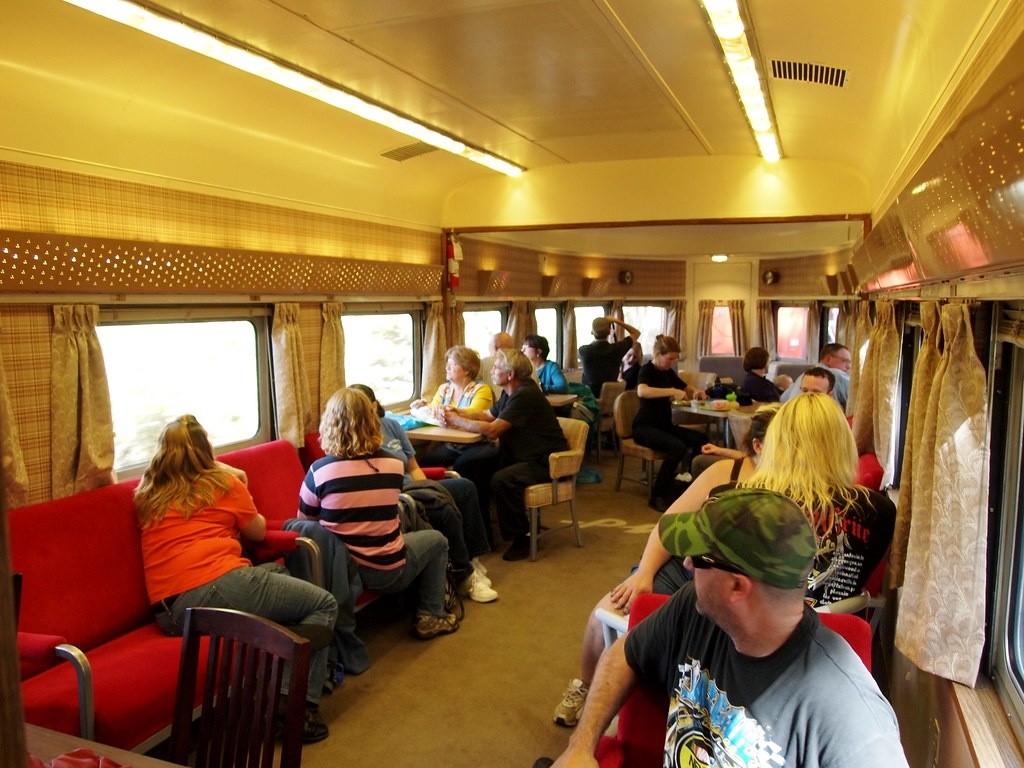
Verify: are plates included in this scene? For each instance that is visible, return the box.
[672,400,691,406]
[699,405,740,410]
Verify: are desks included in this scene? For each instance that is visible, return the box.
[402,422,486,464]
[677,398,762,449]
[543,389,577,407]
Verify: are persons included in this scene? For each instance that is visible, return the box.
[133,415,338,742]
[619,343,643,390]
[632,334,710,513]
[692,367,835,482]
[438,348,571,561]
[348,384,498,603]
[742,347,783,402]
[550,488,910,767]
[578,315,641,399]
[409,345,493,468]
[780,343,851,405]
[706,393,896,607]
[521,335,567,393]
[476,332,539,402]
[552,403,782,728]
[297,387,458,639]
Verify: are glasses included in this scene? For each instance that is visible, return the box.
[830,354,851,364]
[689,554,748,575]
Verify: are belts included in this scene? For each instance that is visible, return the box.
[152,595,178,612]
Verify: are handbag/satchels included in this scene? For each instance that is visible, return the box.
[702,383,754,407]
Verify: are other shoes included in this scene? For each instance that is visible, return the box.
[270,706,329,740]
[503,536,543,560]
[648,496,673,510]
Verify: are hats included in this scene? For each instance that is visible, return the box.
[658,488,819,589]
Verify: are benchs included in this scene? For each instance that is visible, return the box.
[6,439,384,753]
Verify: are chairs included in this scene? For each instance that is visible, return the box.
[595,584,888,768]
[167,607,312,768]
[304,357,891,658]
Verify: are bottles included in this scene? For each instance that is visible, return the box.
[726,392,737,401]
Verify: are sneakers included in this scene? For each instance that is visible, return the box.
[415,613,460,638]
[457,570,498,602]
[443,573,464,620]
[469,557,488,575]
[552,679,588,726]
